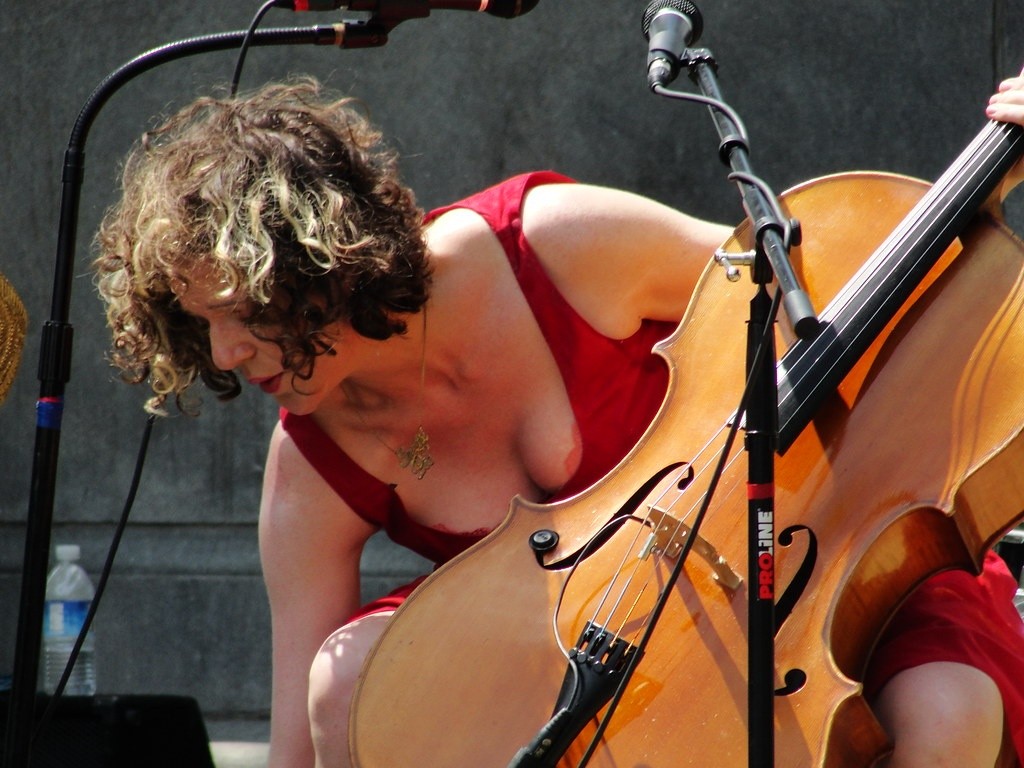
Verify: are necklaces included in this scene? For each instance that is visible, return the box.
[335,276,437,480]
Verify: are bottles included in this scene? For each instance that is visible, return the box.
[44,546,96,695]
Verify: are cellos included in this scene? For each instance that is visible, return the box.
[347,115,1024,767]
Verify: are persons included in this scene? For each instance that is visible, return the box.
[92,60,1024,768]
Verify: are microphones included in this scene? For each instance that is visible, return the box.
[275,0,540,19]
[642,0,705,91]
